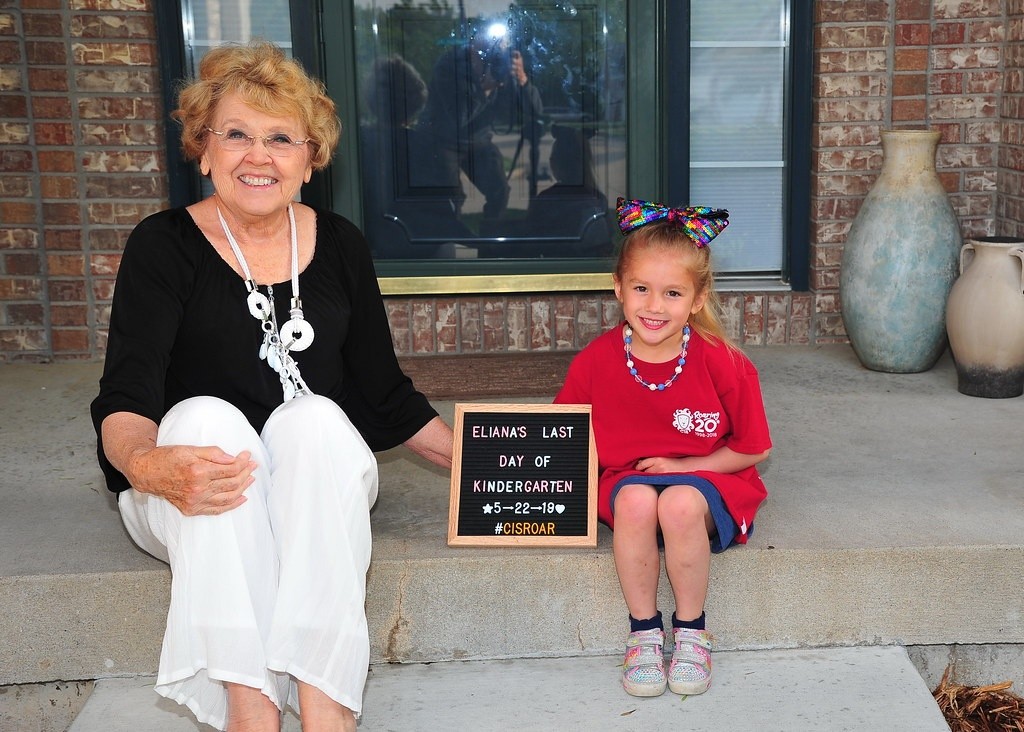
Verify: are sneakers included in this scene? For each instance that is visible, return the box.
[622,628,675,696]
[667,627,712,695]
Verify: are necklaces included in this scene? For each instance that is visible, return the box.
[217,202,314,401]
[624,322,690,391]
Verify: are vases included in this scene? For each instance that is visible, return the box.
[841,130,963,373]
[946,237,1024,398]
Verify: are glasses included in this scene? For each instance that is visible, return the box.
[203,125,311,157]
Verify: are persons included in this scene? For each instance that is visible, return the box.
[548,196,773,695]
[417,12,544,213]
[528,124,606,210]
[359,57,466,219]
[91,43,455,732]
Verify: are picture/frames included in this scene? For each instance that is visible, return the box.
[447,403,597,547]
